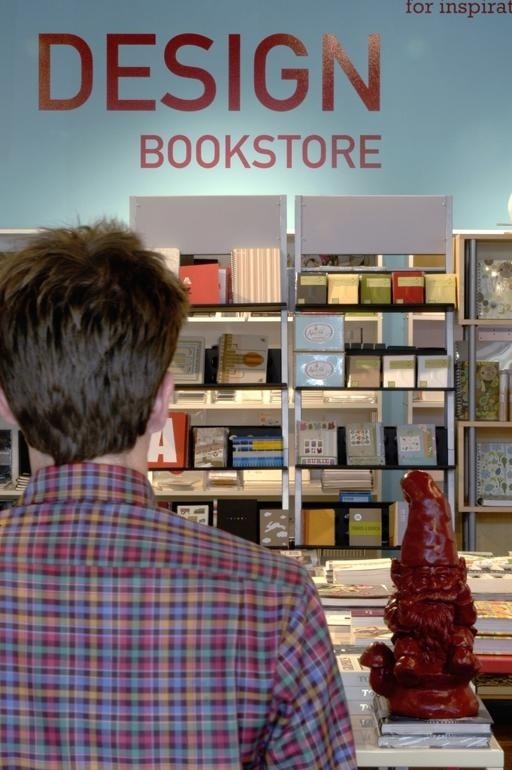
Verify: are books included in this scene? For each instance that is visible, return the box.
[148,334,290,549]
[1,430,55,510]
[455,261,511,506]
[157,246,457,303]
[291,309,450,547]
[291,550,512,749]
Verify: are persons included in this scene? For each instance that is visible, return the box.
[357,464,481,719]
[0,217,361,766]
